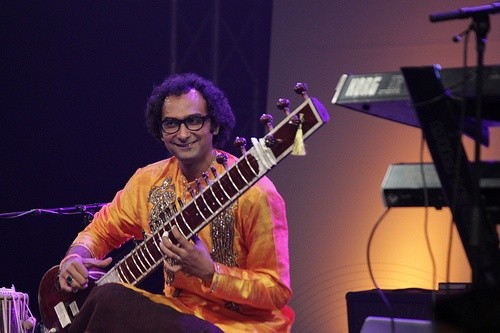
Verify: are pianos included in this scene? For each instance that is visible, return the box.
[331,64,500,132]
[380,160,500,208]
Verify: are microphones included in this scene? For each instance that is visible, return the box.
[429,2,500,22]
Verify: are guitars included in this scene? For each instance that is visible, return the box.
[38,83,329,332]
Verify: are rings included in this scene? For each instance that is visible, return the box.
[174,256,180,263]
[65,274,74,284]
[58,75,295,333]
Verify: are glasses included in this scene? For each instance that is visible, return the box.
[159,114,209,134]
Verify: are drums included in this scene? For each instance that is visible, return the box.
[0,285,36,333]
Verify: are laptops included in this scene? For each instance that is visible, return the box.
[360,316,432,333]
